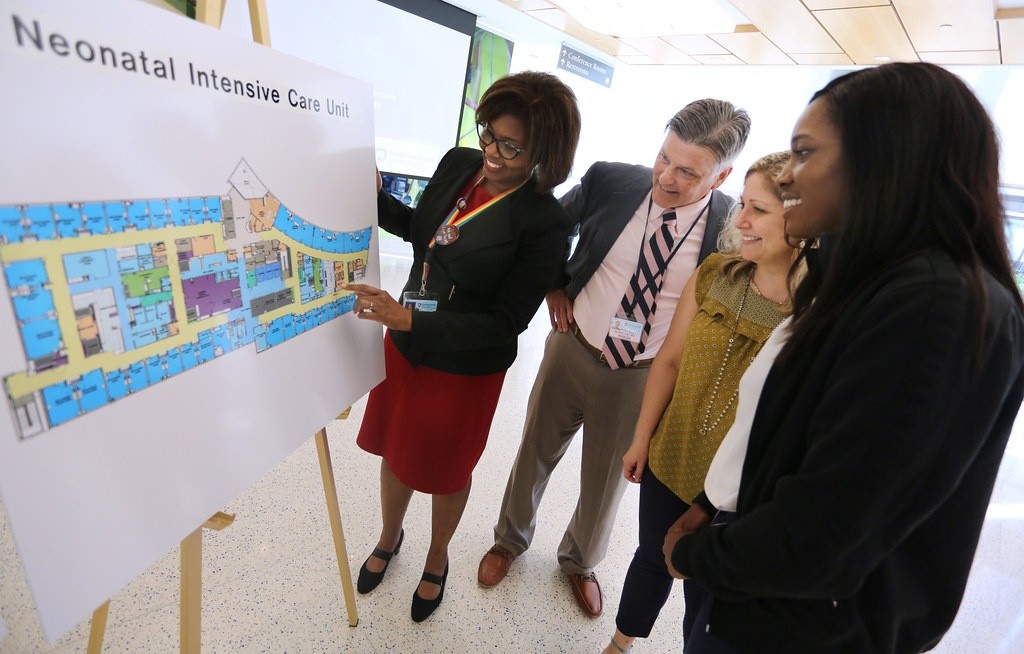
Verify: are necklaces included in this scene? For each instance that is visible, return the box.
[702,265,792,433]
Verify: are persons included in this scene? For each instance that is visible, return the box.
[663,62,1024,654]
[478,98,751,618]
[341,70,581,623]
[601,150,821,654]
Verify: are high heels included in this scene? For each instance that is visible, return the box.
[412,558,449,622]
[357,528,405,593]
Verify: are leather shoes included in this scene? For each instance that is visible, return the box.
[569,571,604,619]
[478,544,515,587]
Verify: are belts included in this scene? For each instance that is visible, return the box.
[571,318,655,367]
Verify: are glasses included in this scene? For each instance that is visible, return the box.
[476,120,526,160]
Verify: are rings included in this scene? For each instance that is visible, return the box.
[369,301,373,310]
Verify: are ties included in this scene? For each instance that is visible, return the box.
[603,208,677,370]
